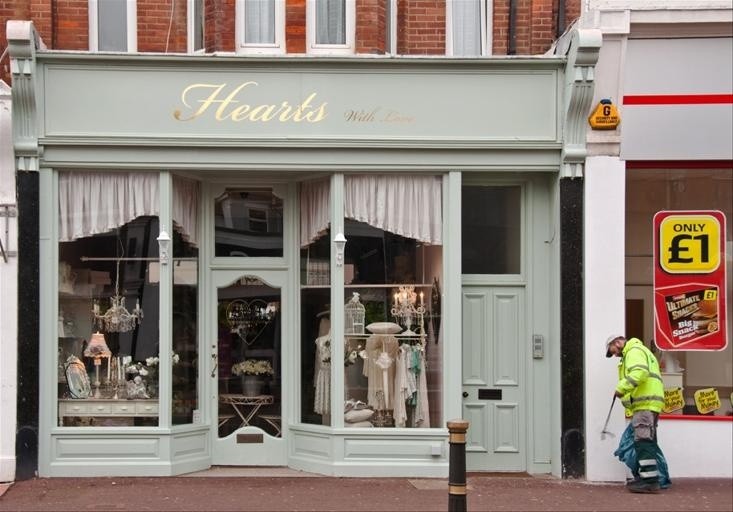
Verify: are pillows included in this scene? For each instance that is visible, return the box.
[366,322,403,333]
[344,409,374,427]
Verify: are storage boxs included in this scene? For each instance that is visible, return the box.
[75,268,111,293]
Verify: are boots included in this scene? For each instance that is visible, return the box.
[629,439,661,493]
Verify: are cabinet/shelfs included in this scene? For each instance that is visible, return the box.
[58,295,92,385]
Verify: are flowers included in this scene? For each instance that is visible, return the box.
[232,360,275,376]
[123,350,179,376]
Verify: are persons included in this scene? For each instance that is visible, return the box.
[605,333,664,494]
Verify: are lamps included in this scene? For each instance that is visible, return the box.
[391,282,426,335]
[84,332,112,399]
[91,230,144,333]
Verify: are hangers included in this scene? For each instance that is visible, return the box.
[397,337,425,353]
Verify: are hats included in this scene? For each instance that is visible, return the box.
[605,333,623,358]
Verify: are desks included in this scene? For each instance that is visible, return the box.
[661,373,684,415]
[219,393,274,427]
[58,398,157,426]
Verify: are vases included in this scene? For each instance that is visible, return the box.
[239,374,266,396]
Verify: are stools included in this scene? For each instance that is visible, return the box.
[218,414,236,429]
[258,413,281,437]
